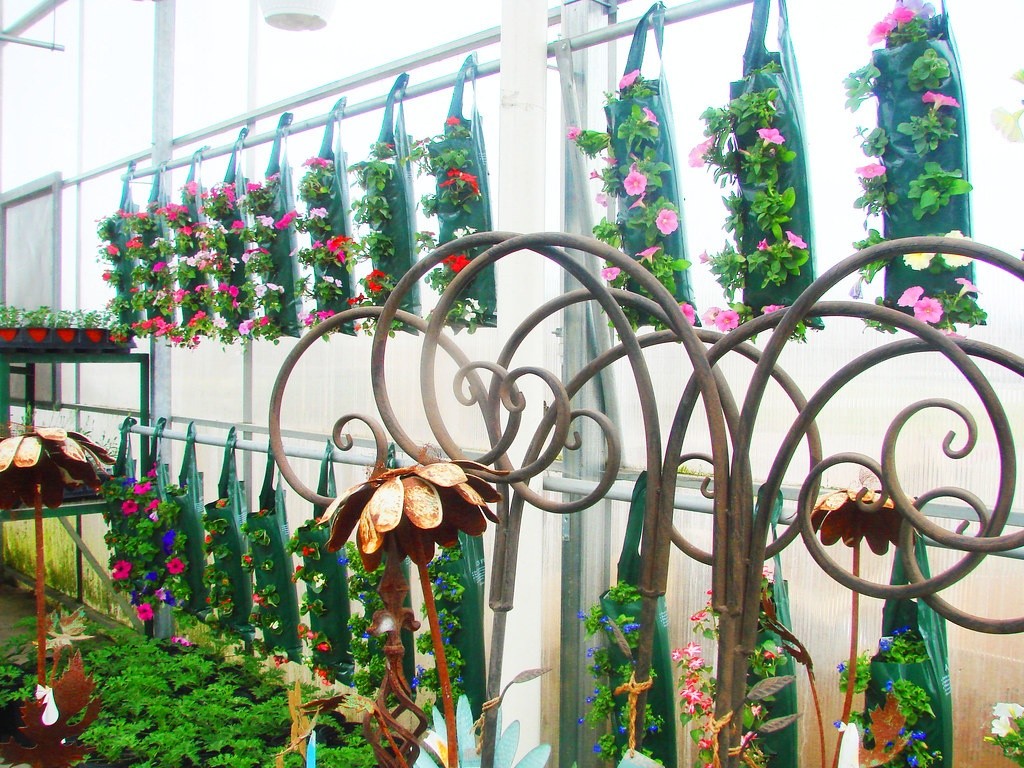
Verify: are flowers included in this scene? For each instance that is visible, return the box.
[1,1,1023,767]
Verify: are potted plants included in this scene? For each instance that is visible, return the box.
[109,315,131,342]
[56,308,81,343]
[0,304,22,340]
[82,308,110,341]
[23,305,56,341]
